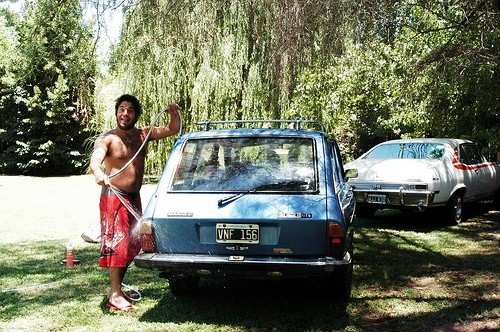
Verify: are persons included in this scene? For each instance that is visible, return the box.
[89,94,182,312]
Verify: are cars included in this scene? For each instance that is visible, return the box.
[343,138,500,224]
[134,119,359,304]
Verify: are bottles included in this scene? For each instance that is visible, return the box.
[67,243,73,267]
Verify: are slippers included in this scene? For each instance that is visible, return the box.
[106,303,138,311]
